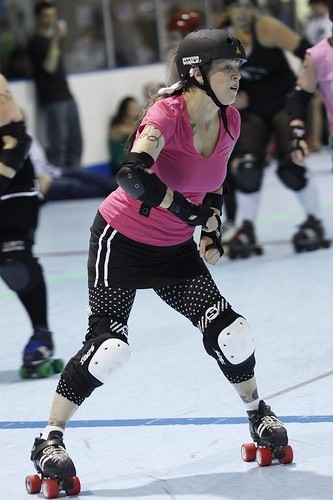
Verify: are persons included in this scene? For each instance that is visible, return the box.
[0,0,85,245]
[30,28,289,479]
[0,72,55,365]
[105,0,333,253]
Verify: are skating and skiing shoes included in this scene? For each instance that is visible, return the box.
[227,220,263,260]
[240,397,294,467]
[19,327,66,377]
[23,427,82,498]
[293,214,332,252]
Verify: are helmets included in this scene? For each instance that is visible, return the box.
[167,10,200,35]
[177,28,249,80]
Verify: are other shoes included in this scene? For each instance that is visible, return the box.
[220,219,237,245]
